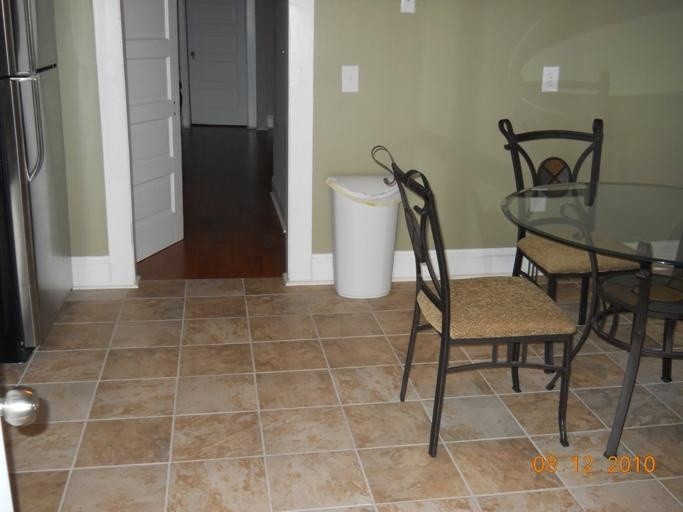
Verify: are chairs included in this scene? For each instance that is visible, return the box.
[499,118,644,373]
[371,145,578,457]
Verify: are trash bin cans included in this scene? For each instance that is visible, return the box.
[326,175,402,299]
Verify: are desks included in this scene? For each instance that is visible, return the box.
[503,183,683,459]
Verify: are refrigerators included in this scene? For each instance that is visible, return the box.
[0,0,74,364]
